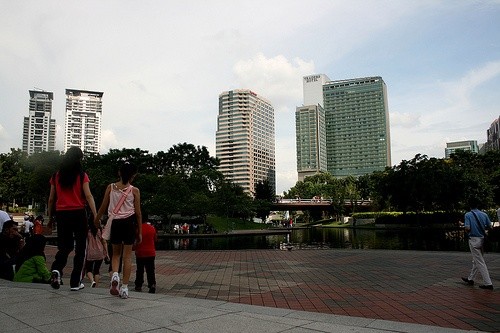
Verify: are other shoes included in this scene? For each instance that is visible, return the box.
[135,287,141,291]
[479,285,493,290]
[51,270,60,289]
[462,277,474,285]
[71,283,84,291]
[91,281,96,288]
[149,286,155,294]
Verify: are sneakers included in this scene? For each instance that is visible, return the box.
[110,277,119,295]
[119,286,128,299]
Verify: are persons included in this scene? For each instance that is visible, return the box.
[131,212,157,292]
[492,204,500,227]
[0,220,24,282]
[276,218,293,227]
[280,196,283,203]
[48,146,98,290]
[94,161,142,298]
[13,234,51,284]
[459,197,494,290]
[296,197,299,203]
[0,197,12,232]
[174,223,199,234]
[312,196,320,203]
[202,222,210,233]
[107,241,122,281]
[353,217,356,226]
[21,214,44,236]
[86,215,108,288]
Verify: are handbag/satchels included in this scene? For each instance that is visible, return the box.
[102,216,113,240]
[483,236,488,253]
